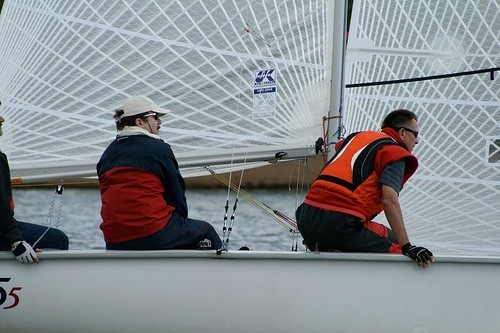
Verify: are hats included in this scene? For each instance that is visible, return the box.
[115,95,171,121]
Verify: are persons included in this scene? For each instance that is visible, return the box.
[95,93,251,253]
[0,100,72,266]
[292,107,437,271]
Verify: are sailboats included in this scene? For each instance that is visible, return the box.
[1,0,500,332]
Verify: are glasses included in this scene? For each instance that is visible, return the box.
[141,113,160,121]
[397,126,419,138]
